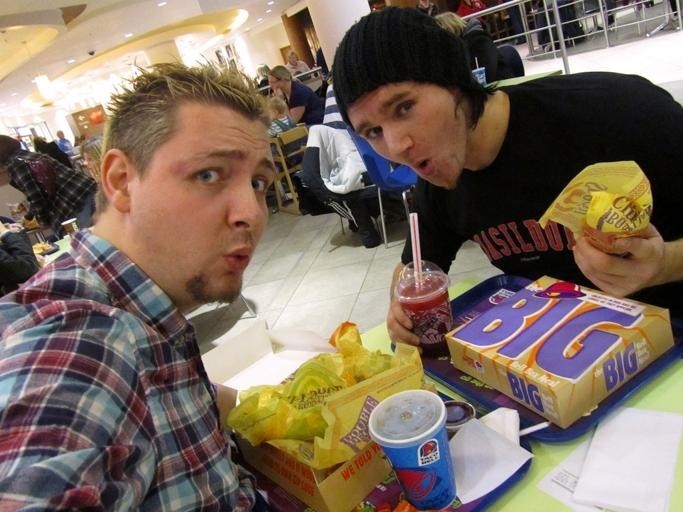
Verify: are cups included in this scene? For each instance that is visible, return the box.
[368,389,457,512]
[61,218,81,239]
[394,260,455,359]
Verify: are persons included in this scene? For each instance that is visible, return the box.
[0,61,278,511]
[508,6,527,45]
[316,48,329,81]
[33,136,74,169]
[0,222,39,298]
[285,51,311,81]
[57,130,73,156]
[332,7,683,356]
[434,12,500,85]
[33,135,46,152]
[75,132,103,178]
[323,65,347,129]
[267,65,323,125]
[267,97,302,171]
[0,135,97,240]
[17,135,31,152]
[254,64,274,96]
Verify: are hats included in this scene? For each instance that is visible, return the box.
[327,5,473,130]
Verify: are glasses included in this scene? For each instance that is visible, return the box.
[269,80,282,85]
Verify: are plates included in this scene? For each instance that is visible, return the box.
[32,242,59,256]
[391,275,679,444]
[436,389,532,510]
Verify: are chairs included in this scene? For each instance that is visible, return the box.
[297,124,394,249]
[269,122,309,215]
[350,129,421,241]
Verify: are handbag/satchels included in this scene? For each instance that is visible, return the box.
[13,155,58,205]
